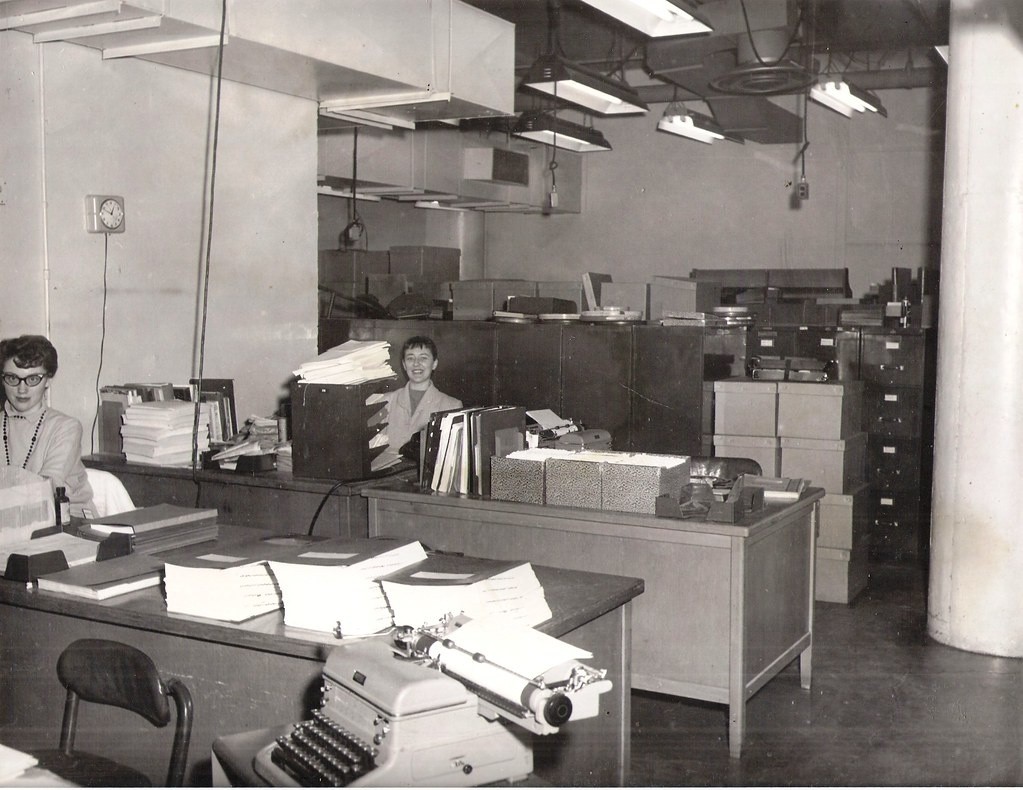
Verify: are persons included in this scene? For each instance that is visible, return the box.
[1,335,99,526]
[380,335,463,459]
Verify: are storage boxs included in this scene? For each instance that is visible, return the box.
[650,274,722,320]
[703,376,873,604]
[0,476,56,545]
[723,298,843,327]
[451,279,589,320]
[491,446,692,514]
[317,246,461,317]
[601,281,650,321]
[510,297,577,314]
[289,379,397,480]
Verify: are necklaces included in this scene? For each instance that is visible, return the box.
[3,409,48,469]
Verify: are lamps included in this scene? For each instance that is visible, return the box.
[809,45,888,119]
[511,92,613,152]
[522,0,650,114]
[657,84,745,146]
[581,0,715,38]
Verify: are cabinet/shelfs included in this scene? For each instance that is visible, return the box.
[81,453,431,539]
[318,318,753,458]
[362,473,826,759]
[0,0,516,122]
[750,329,938,560]
[318,115,581,214]
[0,516,646,788]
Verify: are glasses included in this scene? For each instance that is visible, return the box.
[1,372,47,387]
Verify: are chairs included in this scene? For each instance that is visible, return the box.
[19,639,195,788]
[691,456,763,487]
[86,469,136,518]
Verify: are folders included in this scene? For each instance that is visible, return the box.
[83,501,220,534]
[468,405,528,497]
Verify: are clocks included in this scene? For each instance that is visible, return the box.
[86,196,125,233]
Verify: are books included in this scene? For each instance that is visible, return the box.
[165,536,318,625]
[100,380,293,474]
[383,555,552,638]
[420,404,534,504]
[268,539,432,637]
[37,553,165,601]
[77,502,219,556]
[738,473,805,500]
